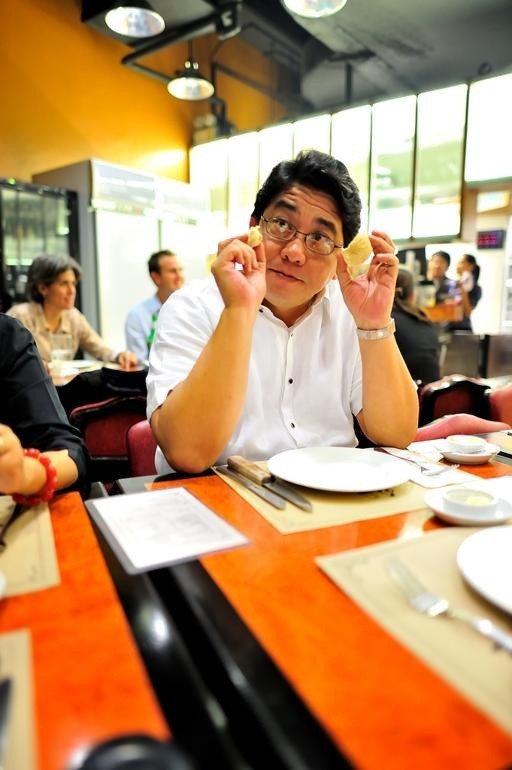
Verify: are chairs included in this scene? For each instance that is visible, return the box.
[125,419,159,479]
[413,373,511,441]
[70,394,147,460]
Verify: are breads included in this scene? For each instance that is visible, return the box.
[247,226,262,247]
[342,232,372,266]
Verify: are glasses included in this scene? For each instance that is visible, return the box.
[260,215,345,255]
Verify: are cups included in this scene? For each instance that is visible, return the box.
[51,333,76,379]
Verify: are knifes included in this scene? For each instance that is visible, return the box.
[215,465,287,510]
[227,454,315,514]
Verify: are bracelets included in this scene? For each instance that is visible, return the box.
[12,445,57,507]
[357,315,398,340]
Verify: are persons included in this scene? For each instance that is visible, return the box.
[450,254,481,330]
[354,267,441,449]
[125,250,184,362]
[3,253,139,385]
[144,148,421,474]
[417,250,457,332]
[1,315,93,505]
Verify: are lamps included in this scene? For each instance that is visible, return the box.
[166,39,215,101]
[280,0,346,19]
[103,0,166,39]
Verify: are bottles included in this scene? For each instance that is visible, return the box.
[147,311,158,359]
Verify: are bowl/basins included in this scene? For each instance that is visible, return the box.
[443,487,500,513]
[446,434,489,456]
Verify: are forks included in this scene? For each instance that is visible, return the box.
[384,554,512,660]
[374,446,461,478]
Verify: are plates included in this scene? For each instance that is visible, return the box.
[452,520,512,619]
[267,445,414,494]
[48,358,95,369]
[419,481,511,526]
[434,443,501,464]
